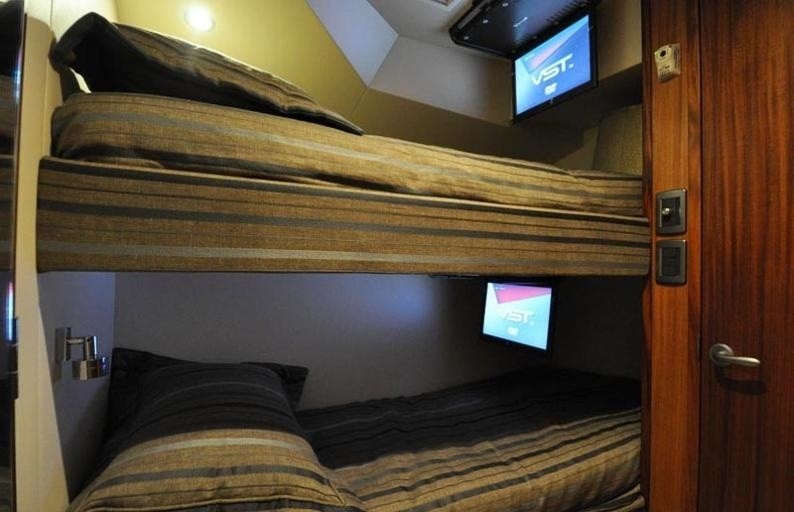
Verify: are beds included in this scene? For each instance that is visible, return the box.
[2,14,650,511]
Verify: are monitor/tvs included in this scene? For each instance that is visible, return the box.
[475,279,559,356]
[511,11,600,125]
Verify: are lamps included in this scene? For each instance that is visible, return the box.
[56,326,109,379]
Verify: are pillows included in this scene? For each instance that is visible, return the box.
[66,348,367,512]
[0,0,24,77]
[50,10,367,137]
